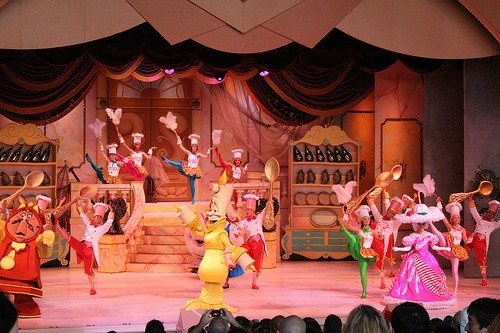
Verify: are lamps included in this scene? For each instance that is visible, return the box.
[165,68,175,74]
[213,71,226,81]
[258,68,270,77]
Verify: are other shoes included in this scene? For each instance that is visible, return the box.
[380,284,386,289]
[481,280,487,286]
[223,283,229,288]
[51,214,55,225]
[90,289,96,295]
[162,156,167,163]
[252,283,258,289]
[388,273,395,277]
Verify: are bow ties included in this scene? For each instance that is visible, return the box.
[90,220,98,228]
[247,216,256,221]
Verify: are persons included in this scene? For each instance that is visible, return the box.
[343,190,411,289]
[144,296,499,333]
[190,208,245,288]
[382,186,414,278]
[115,126,158,181]
[232,194,270,289]
[336,206,378,299]
[86,145,133,184]
[158,135,213,205]
[383,204,456,310]
[215,147,250,210]
[428,202,475,298]
[466,191,500,286]
[51,194,115,295]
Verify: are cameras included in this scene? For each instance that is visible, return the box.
[209,310,223,317]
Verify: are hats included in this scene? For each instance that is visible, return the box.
[389,196,404,214]
[93,203,108,218]
[446,202,462,218]
[131,133,144,145]
[353,205,371,223]
[394,204,445,224]
[488,200,500,213]
[243,194,259,212]
[188,134,200,145]
[106,144,118,154]
[231,148,244,158]
[402,193,413,209]
[35,194,51,210]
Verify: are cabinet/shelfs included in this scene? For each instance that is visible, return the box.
[0,122,59,210]
[281,125,361,259]
